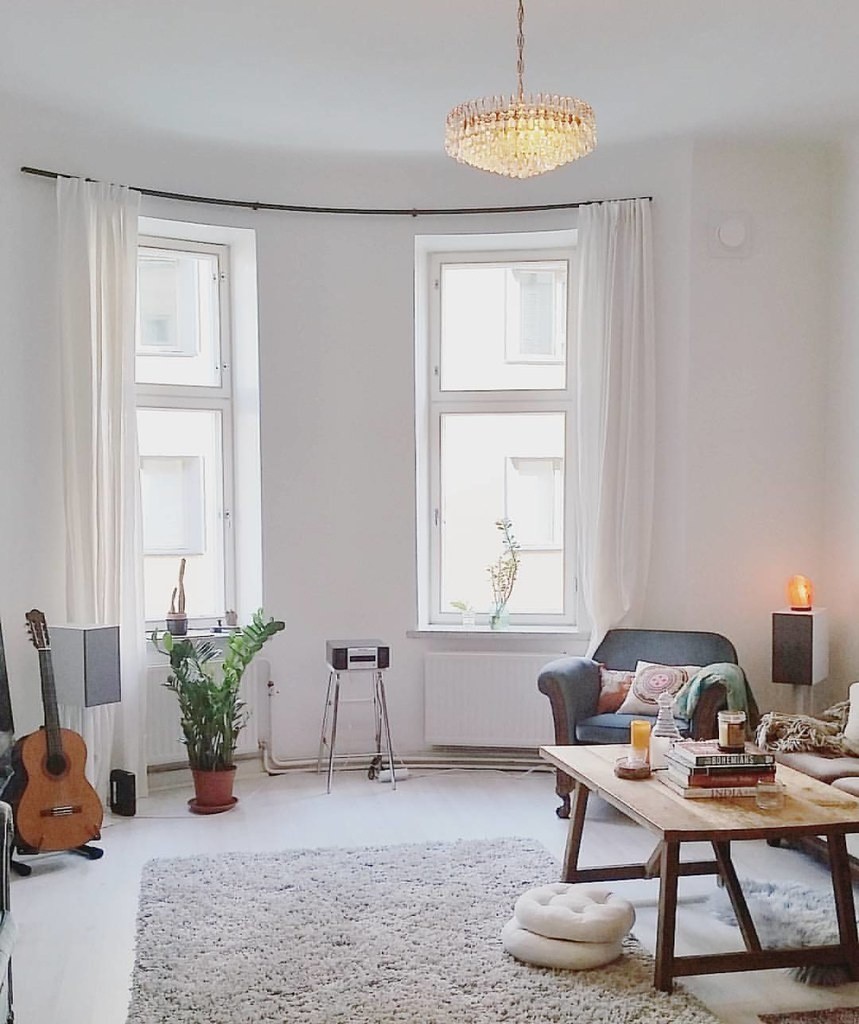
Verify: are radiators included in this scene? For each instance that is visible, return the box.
[423,651,566,747]
[146,660,259,765]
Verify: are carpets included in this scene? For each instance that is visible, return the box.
[128,837,720,1024]
[756,1005,859,1024]
[714,880,859,987]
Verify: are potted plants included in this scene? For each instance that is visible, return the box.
[487,517,521,630]
[167,558,188,634]
[152,607,286,813]
[450,600,475,627]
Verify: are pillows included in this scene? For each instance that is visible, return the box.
[616,660,702,719]
[597,667,636,714]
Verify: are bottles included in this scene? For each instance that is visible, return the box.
[650,690,680,769]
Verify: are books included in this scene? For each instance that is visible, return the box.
[653,742,783,798]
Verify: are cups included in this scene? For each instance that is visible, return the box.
[718,710,746,752]
[627,746,648,768]
[757,778,784,810]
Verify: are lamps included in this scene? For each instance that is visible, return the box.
[786,575,814,612]
[444,0,598,179]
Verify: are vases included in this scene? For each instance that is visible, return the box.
[223,612,238,627]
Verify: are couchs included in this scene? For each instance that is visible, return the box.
[537,628,758,818]
[755,682,859,887]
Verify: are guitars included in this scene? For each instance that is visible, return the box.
[13,609,103,851]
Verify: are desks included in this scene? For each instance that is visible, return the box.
[314,660,395,793]
[539,739,859,995]
